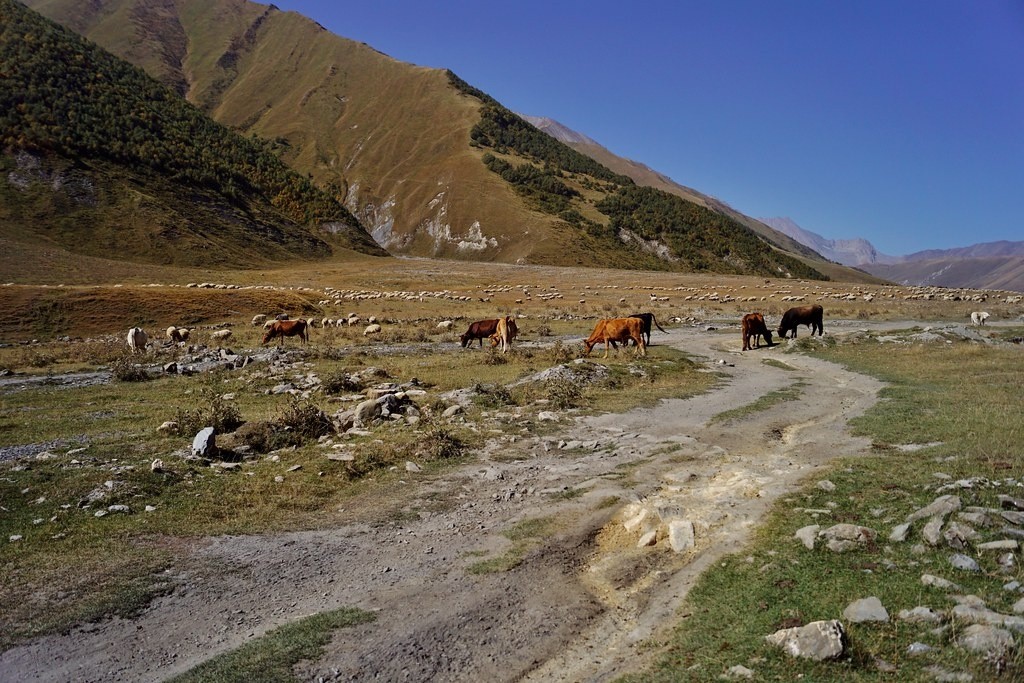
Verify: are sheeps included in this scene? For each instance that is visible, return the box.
[252,313,266,326]
[970,311,990,327]
[367,316,377,323]
[166,326,190,341]
[187,282,470,305]
[127,326,147,353]
[211,329,232,340]
[274,313,290,320]
[307,318,315,325]
[436,320,455,331]
[476,284,563,304]
[364,324,381,336]
[322,313,361,327]
[571,280,1022,304]
[263,320,279,330]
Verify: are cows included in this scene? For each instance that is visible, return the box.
[262,319,309,344]
[777,305,823,336]
[742,312,774,351]
[459,316,520,353]
[582,313,670,360]
